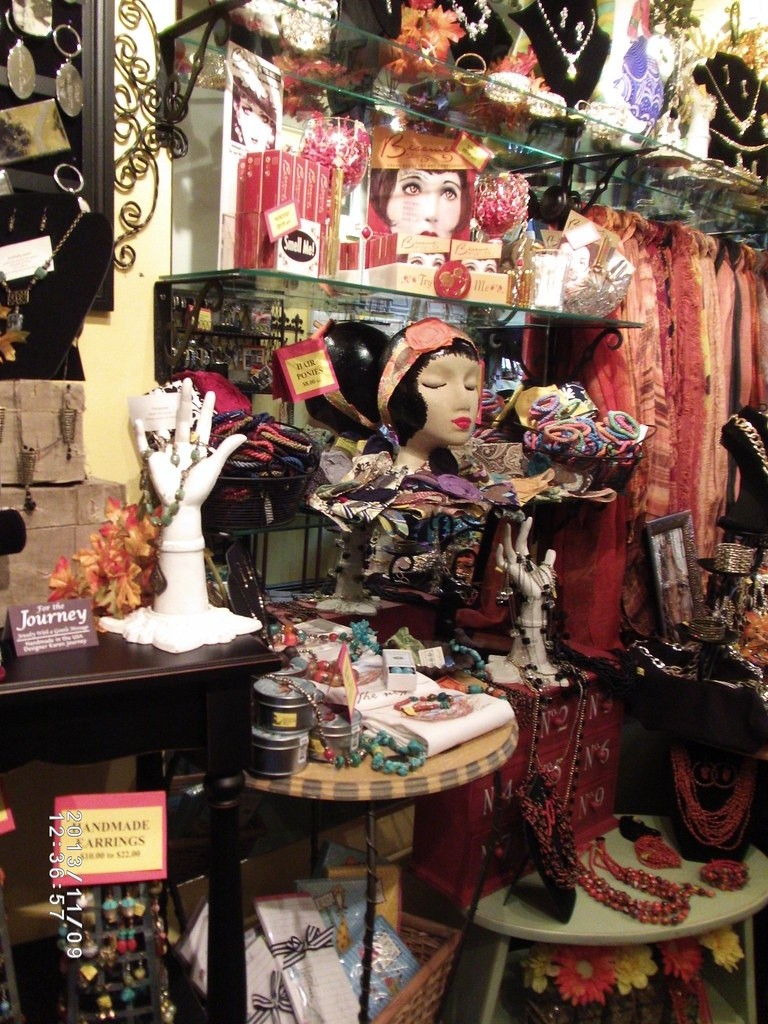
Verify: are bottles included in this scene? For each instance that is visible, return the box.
[753,550,768,615]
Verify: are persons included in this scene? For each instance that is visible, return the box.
[721,405,768,532]
[511,0,611,112]
[436,1,516,75]
[303,319,389,451]
[134,379,248,614]
[695,54,768,181]
[493,517,558,668]
[228,75,276,151]
[376,317,485,475]
[1,193,113,382]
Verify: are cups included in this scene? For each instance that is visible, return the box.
[708,543,757,643]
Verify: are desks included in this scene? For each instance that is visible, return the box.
[438,815,768,1024]
[0,619,289,1022]
[239,671,520,1023]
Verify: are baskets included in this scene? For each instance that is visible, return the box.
[371,912,462,1024]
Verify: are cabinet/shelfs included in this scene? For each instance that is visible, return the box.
[153,0,768,537]
[272,592,626,908]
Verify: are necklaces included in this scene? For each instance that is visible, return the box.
[0,0,768,1023]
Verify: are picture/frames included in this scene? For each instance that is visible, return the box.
[640,509,707,639]
